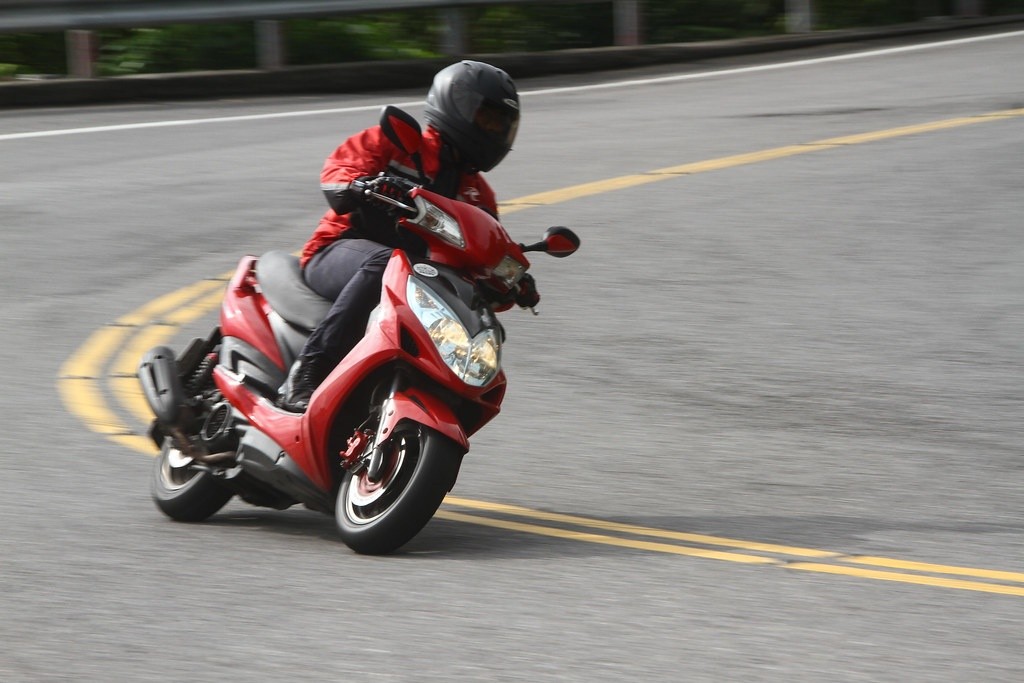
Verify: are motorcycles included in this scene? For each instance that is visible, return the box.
[134,105,582,556]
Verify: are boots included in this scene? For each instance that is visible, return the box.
[275,358,314,409]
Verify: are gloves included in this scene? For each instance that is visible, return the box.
[500,272,540,307]
[351,175,410,224]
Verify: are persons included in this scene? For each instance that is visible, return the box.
[274,60,521,414]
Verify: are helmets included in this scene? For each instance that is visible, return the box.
[424,60,520,172]
